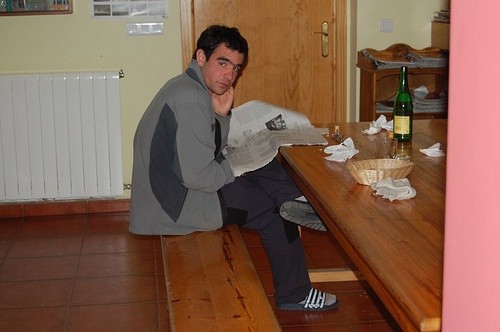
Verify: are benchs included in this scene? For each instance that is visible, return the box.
[161,222,282,332]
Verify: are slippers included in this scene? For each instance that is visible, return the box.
[276,288,339,311]
[279,199,329,232]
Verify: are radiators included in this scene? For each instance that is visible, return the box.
[0,69,131,201]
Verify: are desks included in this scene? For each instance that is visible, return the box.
[277,117,445,332]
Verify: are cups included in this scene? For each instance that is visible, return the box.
[374,137,398,159]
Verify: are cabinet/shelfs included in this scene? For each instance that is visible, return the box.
[356,43,449,122]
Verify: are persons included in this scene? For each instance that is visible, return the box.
[127,24,339,312]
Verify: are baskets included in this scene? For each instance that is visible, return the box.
[344,158,414,186]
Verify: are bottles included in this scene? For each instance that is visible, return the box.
[392,66,413,143]
